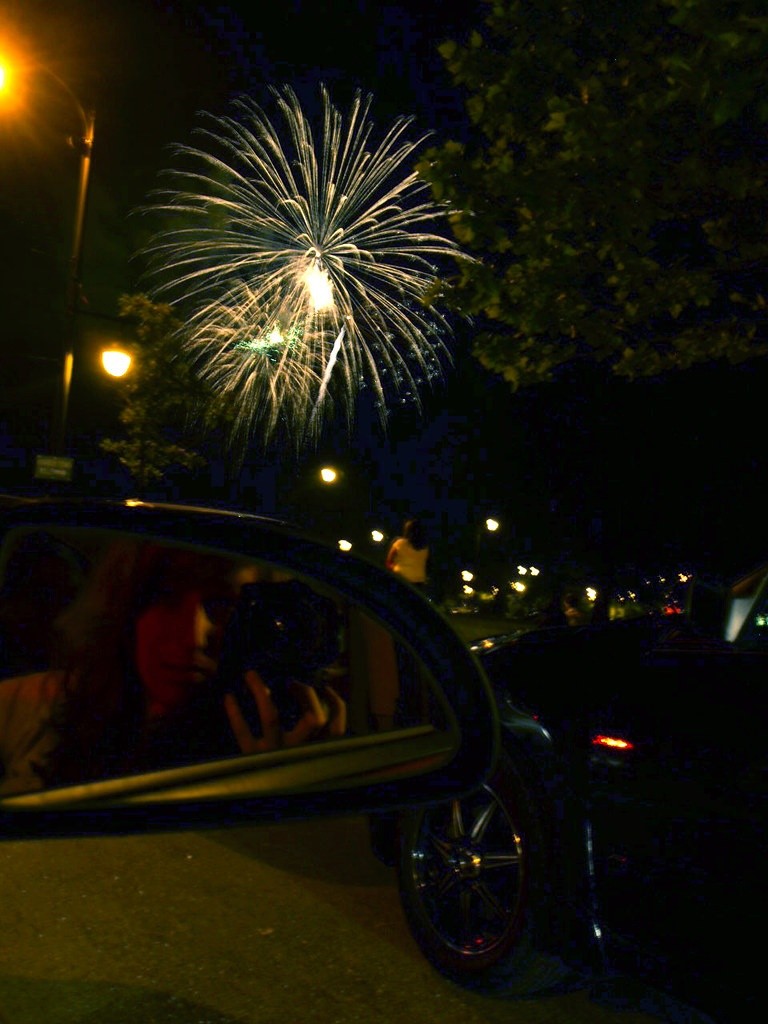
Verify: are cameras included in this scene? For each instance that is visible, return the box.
[211,579,349,731]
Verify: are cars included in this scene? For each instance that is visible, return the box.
[371,560,768,1005]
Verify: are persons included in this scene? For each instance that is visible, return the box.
[0,540,350,797]
[563,592,583,626]
[387,518,429,589]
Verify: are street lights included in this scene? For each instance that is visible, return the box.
[0,55,94,456]
[286,468,338,522]
[473,518,499,592]
